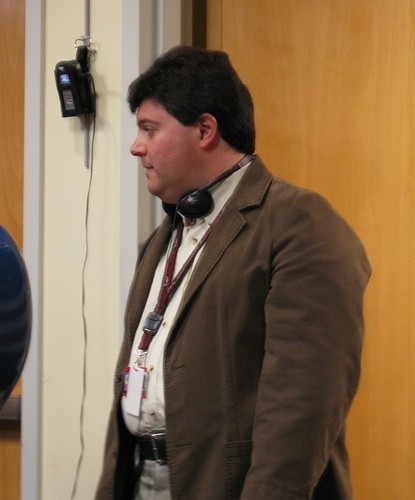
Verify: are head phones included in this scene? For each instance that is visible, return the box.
[162,154,253,221]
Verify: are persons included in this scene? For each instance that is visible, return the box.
[0,224,32,410]
[94,44,373,500]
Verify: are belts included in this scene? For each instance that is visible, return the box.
[126,432,166,464]
